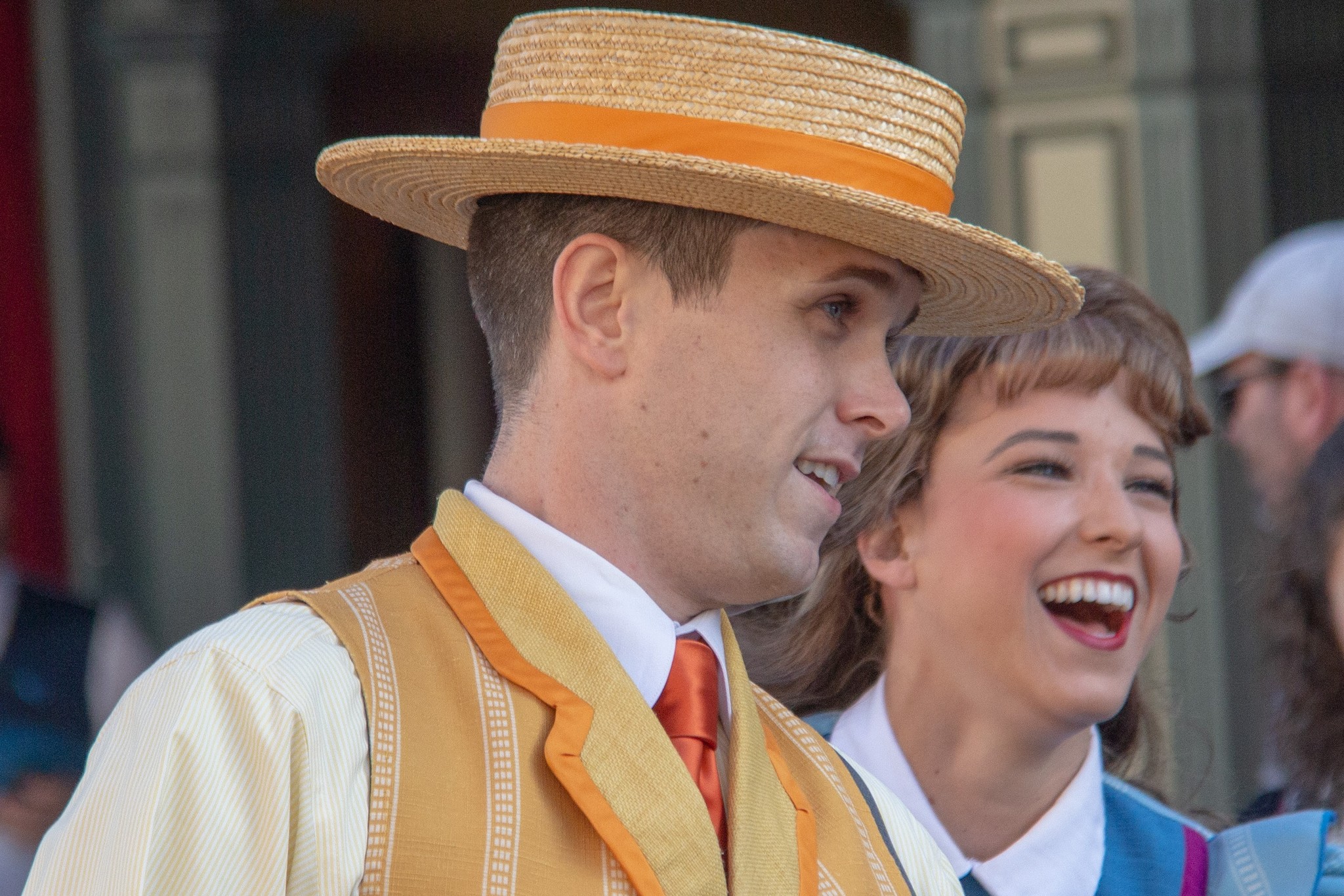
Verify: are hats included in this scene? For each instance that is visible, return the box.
[1184,222,1343,388]
[317,9,1090,337]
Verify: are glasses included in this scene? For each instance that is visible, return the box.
[1216,356,1293,426]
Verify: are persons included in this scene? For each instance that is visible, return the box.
[742,267,1218,895]
[0,436,154,896]
[1200,422,1343,895]
[23,9,1079,896]
[1183,218,1343,537]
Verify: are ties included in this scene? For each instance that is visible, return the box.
[652,634,727,859]
